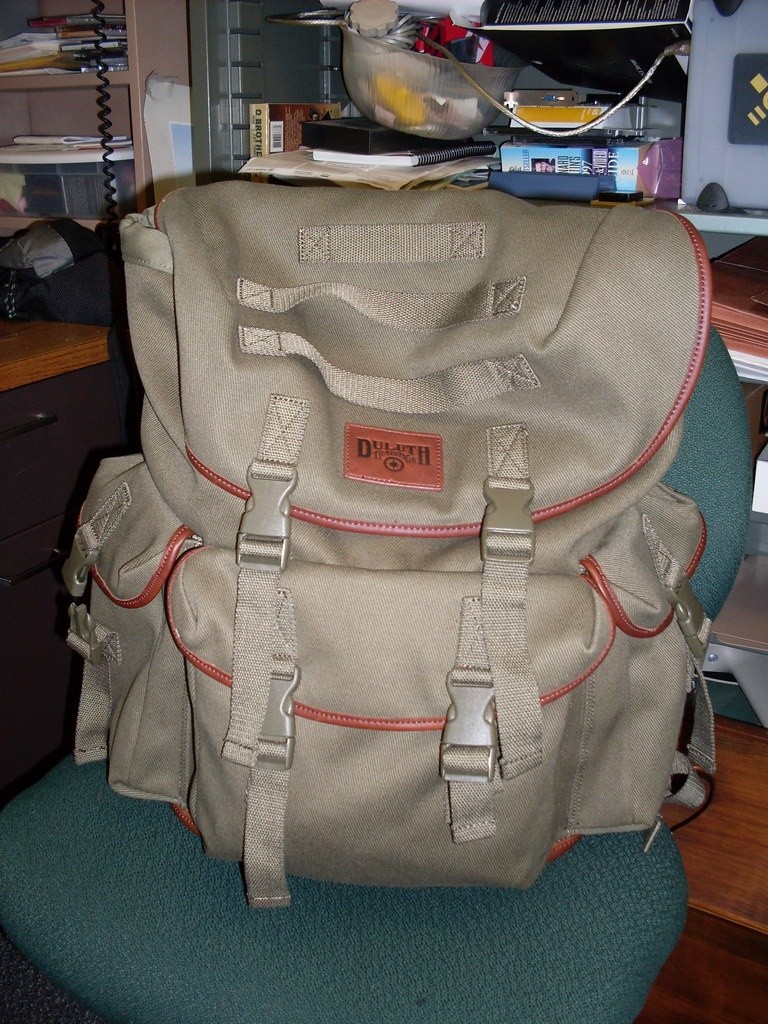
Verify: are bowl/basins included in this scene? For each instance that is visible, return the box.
[341,23,530,140]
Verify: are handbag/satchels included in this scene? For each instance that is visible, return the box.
[0,219,125,326]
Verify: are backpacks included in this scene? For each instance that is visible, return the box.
[68,181,715,908]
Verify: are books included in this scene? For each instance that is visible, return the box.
[304,139,497,166]
[1,14,128,76]
[2,133,132,150]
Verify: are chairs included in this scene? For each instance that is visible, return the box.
[0,327,755,1024]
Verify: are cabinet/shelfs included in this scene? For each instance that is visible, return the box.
[0,0,196,236]
[665,236,768,937]
[0,316,138,806]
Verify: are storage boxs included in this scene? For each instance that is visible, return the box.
[448,0,694,93]
[500,138,681,201]
[249,101,343,184]
